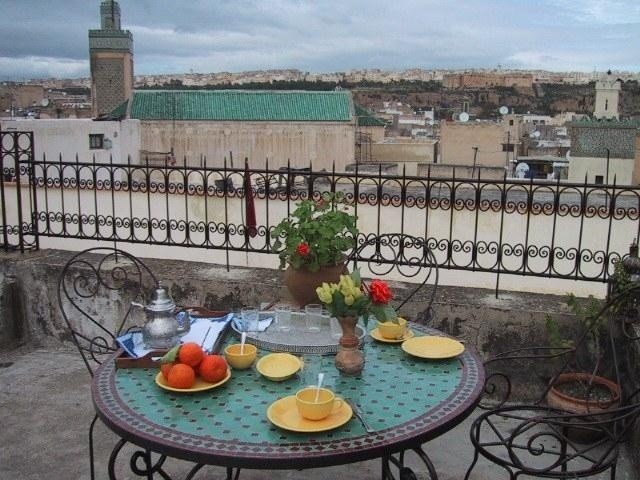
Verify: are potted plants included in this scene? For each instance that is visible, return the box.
[545,254,640,443]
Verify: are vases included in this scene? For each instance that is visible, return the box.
[281,255,348,308]
[333,315,368,374]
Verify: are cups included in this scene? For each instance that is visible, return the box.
[224,344,257,370]
[241,307,259,332]
[377,318,410,339]
[276,305,291,331]
[305,304,322,332]
[330,318,343,341]
[302,353,324,389]
[295,388,342,421]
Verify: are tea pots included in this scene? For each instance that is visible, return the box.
[129,281,190,351]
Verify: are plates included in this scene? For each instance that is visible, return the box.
[267,396,352,433]
[370,328,414,343]
[155,367,232,393]
[402,336,465,359]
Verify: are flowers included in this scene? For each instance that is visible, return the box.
[315,265,396,327]
[265,189,359,271]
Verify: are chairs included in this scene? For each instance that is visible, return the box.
[463,286,640,480]
[57,246,167,480]
[338,232,440,325]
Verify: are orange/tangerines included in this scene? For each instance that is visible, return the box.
[159,343,227,389]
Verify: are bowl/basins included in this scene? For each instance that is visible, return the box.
[256,353,301,381]
